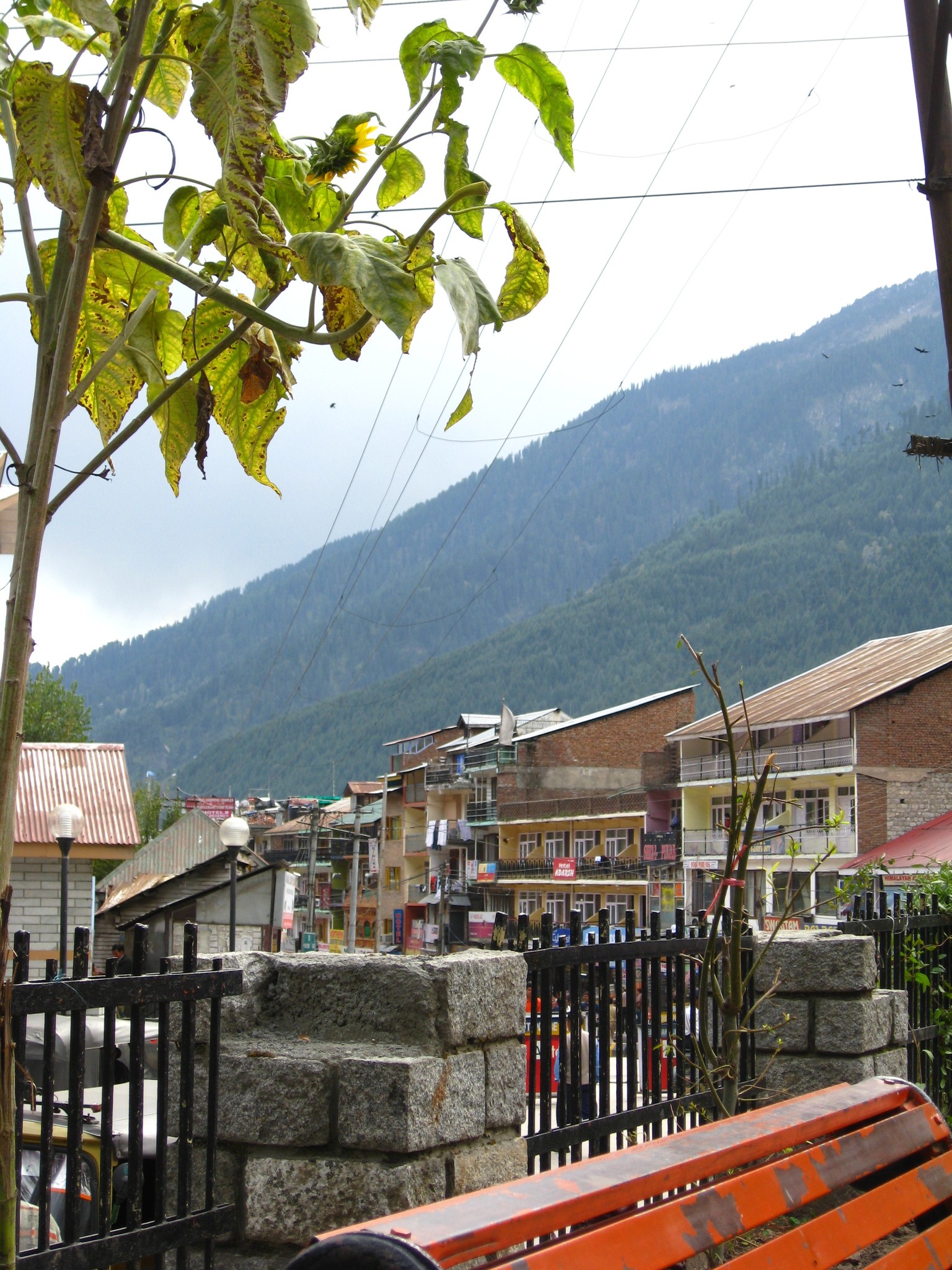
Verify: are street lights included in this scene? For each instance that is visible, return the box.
[218,816,250,952]
[47,803,86,978]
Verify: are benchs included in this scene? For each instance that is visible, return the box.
[286,1076,952,1270]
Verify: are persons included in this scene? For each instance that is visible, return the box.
[92,963,100,976]
[112,944,132,1020]
[526,979,699,1127]
